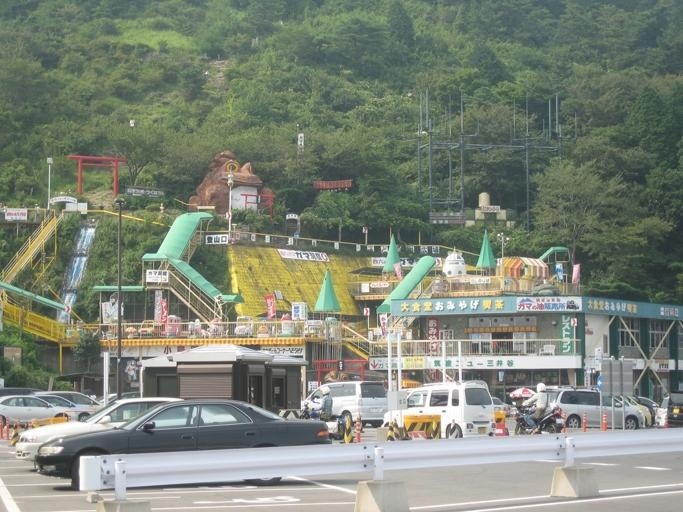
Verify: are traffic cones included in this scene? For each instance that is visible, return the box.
[384,419,403,442]
[6,421,19,448]
[339,416,356,444]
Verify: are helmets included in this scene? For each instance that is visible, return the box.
[537,383,546,392]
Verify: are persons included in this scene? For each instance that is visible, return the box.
[521,382,547,436]
[316,385,332,416]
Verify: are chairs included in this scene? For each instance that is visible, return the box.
[193,416,204,426]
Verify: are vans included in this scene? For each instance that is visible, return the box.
[383,380,493,439]
[302,379,387,426]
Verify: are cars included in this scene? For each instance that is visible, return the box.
[544,387,683,430]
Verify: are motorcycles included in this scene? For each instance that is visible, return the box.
[514,405,567,437]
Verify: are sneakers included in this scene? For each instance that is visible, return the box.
[531,428,539,435]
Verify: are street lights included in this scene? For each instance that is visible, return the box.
[111,197,126,401]
[226,173,235,241]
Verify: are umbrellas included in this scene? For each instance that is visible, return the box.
[508,387,536,401]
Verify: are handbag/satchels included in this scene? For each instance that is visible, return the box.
[533,408,544,421]
